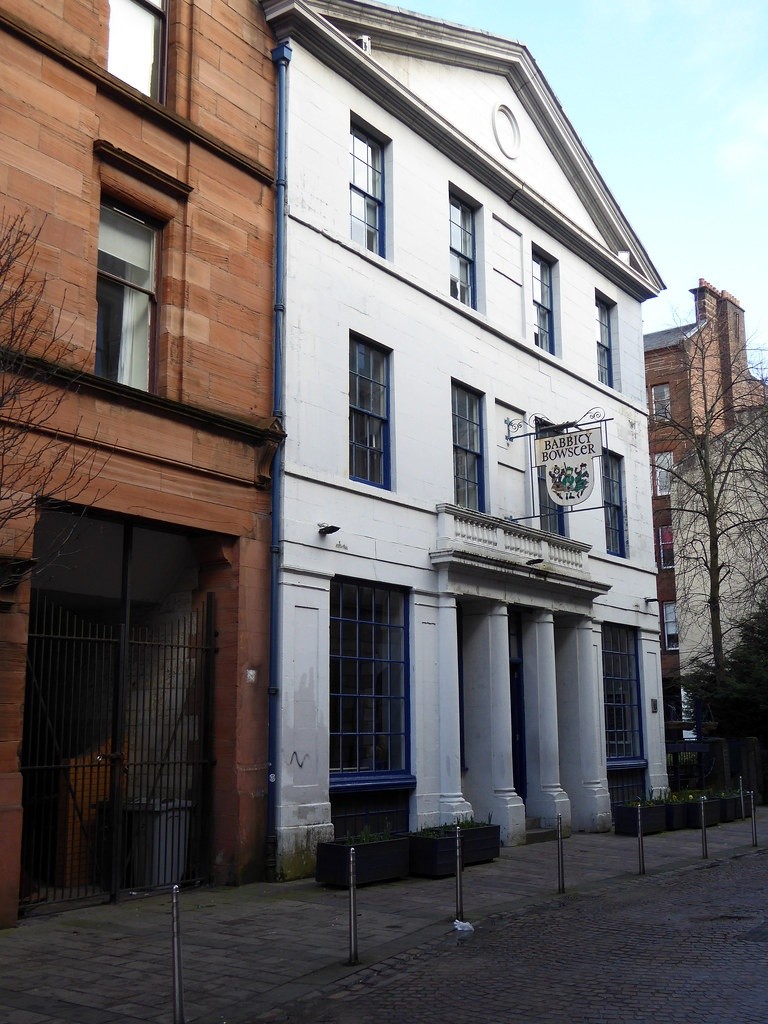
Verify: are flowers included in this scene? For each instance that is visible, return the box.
[653,795,682,802]
[684,793,700,801]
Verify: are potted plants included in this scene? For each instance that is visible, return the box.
[317,834,408,886]
[434,812,501,864]
[685,798,720,827]
[731,790,755,817]
[404,830,462,877]
[713,793,737,822]
[615,801,666,834]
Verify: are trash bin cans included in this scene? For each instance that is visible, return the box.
[90,797,136,890]
[126,797,195,890]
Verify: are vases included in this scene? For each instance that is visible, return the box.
[655,802,686,830]
